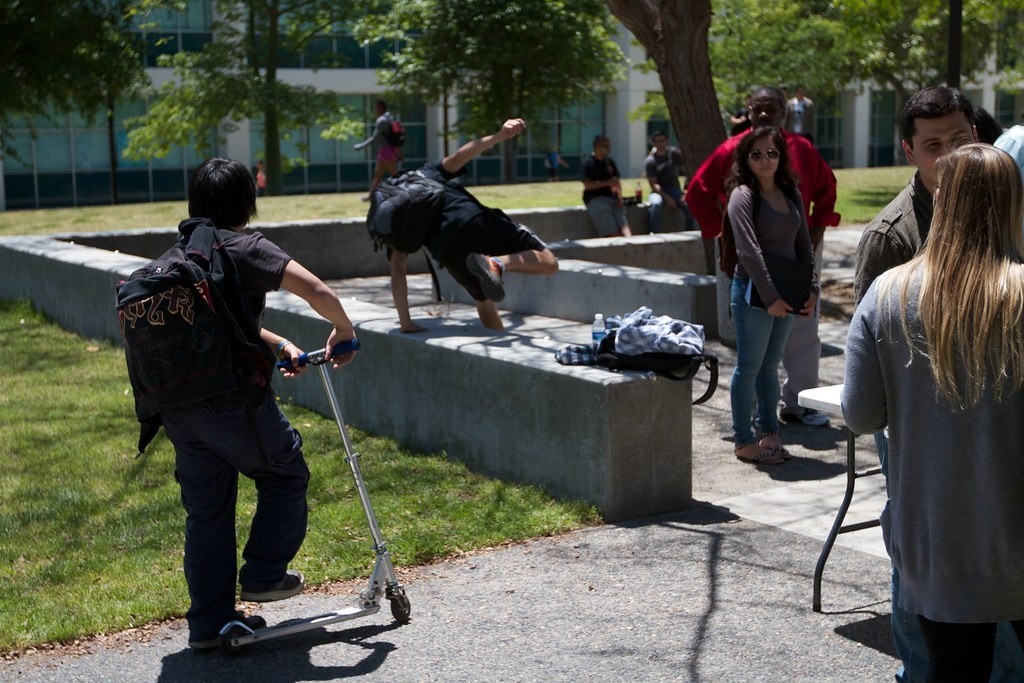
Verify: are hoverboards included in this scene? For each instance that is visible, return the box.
[220,336,414,660]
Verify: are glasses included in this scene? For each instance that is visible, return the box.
[748,149,780,161]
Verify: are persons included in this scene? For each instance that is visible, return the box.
[685,83,840,426]
[841,85,1024,683]
[644,130,697,233]
[353,99,403,202]
[719,125,820,466]
[544,139,569,181]
[581,135,632,238]
[165,157,356,650]
[366,119,558,334]
[253,160,266,197]
[725,84,815,147]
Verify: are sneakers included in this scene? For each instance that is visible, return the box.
[188,611,267,649]
[240,570,304,602]
[780,409,830,425]
[466,253,506,302]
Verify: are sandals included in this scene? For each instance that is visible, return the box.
[757,431,793,459]
[735,439,784,464]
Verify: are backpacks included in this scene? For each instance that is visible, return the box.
[366,171,445,259]
[597,328,720,404]
[386,120,407,148]
[116,217,263,426]
[714,208,738,279]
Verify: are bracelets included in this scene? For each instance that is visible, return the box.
[275,339,292,360]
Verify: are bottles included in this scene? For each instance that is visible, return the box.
[592,314,606,350]
[636,183,641,203]
